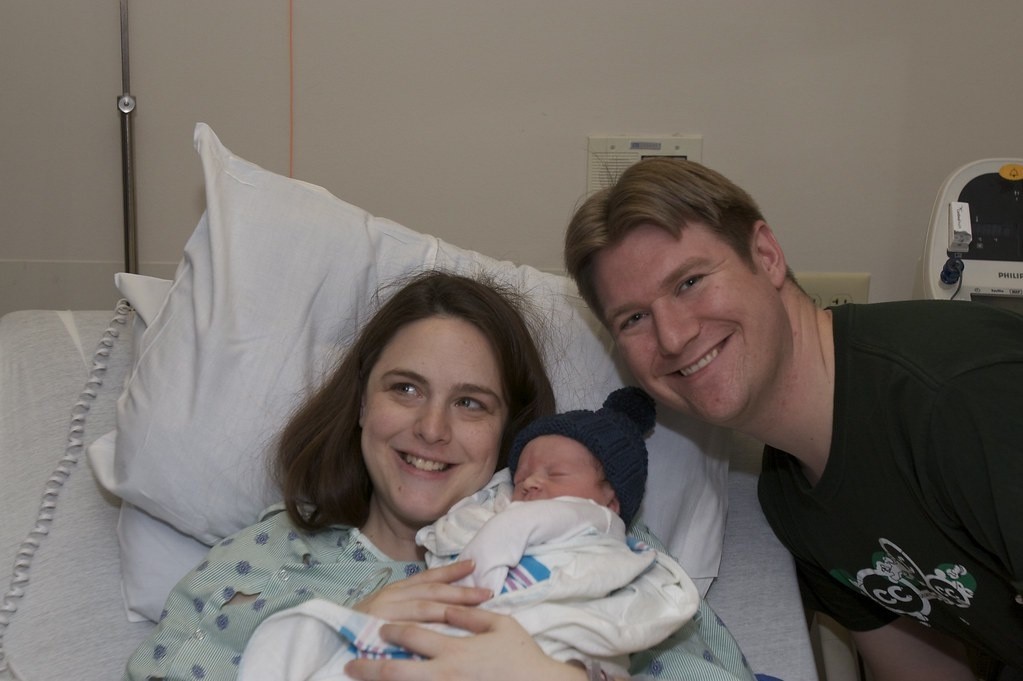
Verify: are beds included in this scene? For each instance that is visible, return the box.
[0,310,819,681]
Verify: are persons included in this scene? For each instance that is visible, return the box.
[237,385,699,681]
[564,157,1023,681]
[128,274,755,681]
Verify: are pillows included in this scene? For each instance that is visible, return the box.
[111,271,209,622]
[86,122,708,583]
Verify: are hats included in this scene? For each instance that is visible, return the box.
[508,386,659,529]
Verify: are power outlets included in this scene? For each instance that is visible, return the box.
[793,268,871,306]
